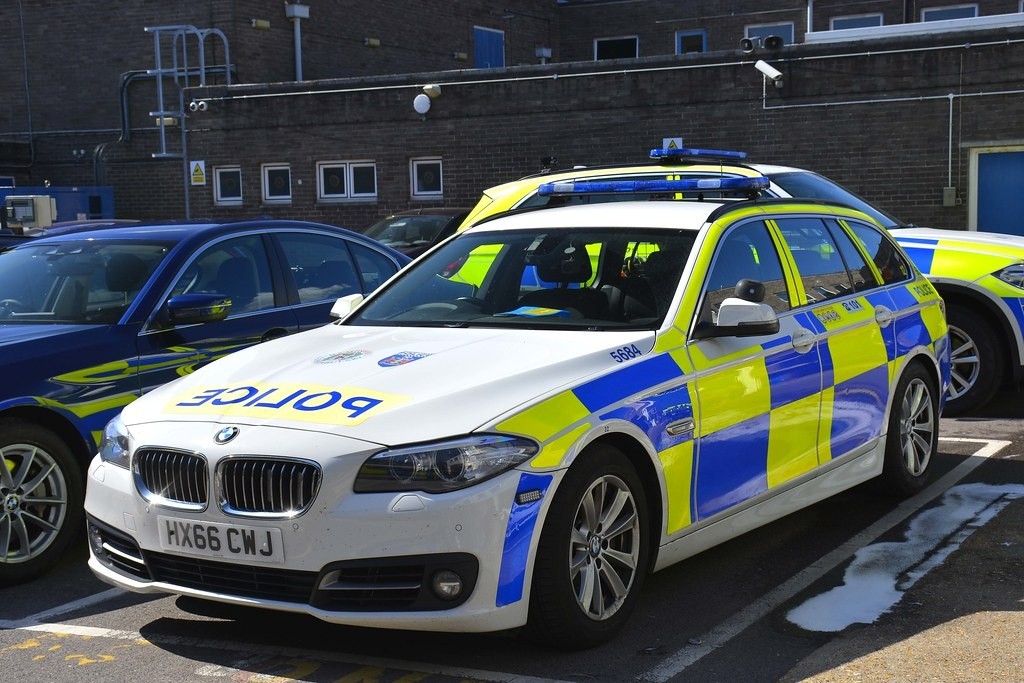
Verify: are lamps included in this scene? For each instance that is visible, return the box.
[422,84,442,99]
[413,94,431,115]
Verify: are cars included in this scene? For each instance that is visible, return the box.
[0,218,415,590]
[359,206,473,261]
[439,146,1024,415]
[80,177,954,646]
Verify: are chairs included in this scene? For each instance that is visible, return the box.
[217,259,272,309]
[624,245,713,324]
[719,239,763,288]
[317,258,357,290]
[106,254,147,292]
[517,240,612,322]
[763,250,828,282]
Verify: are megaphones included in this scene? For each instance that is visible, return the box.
[739,36,761,53]
[763,35,784,51]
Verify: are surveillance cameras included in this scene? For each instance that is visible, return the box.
[189,102,198,111]
[198,102,209,111]
[755,60,783,80]
